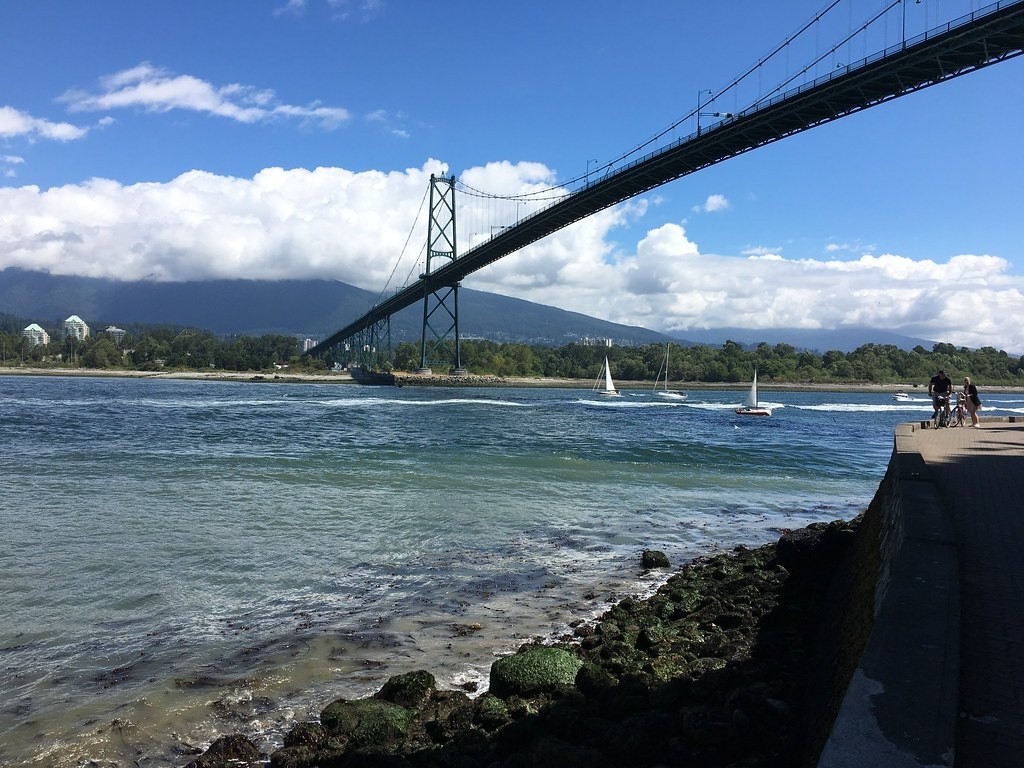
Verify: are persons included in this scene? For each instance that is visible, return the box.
[927,370,953,419]
[963,376,982,428]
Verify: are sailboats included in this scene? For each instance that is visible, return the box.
[651,343,688,400]
[592,355,622,397]
[735,368,772,416]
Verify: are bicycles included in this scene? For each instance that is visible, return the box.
[929,394,951,430]
[949,391,972,428]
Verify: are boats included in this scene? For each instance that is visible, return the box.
[892,393,914,402]
[350,367,395,386]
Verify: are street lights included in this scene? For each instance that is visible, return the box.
[517,202,526,226]
[698,89,712,137]
[587,158,597,189]
[903,0,921,49]
[469,233,477,254]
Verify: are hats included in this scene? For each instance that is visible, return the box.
[939,370,947,375]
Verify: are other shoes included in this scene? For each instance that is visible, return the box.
[944,415,950,421]
[932,411,938,418]
[969,423,981,429]
